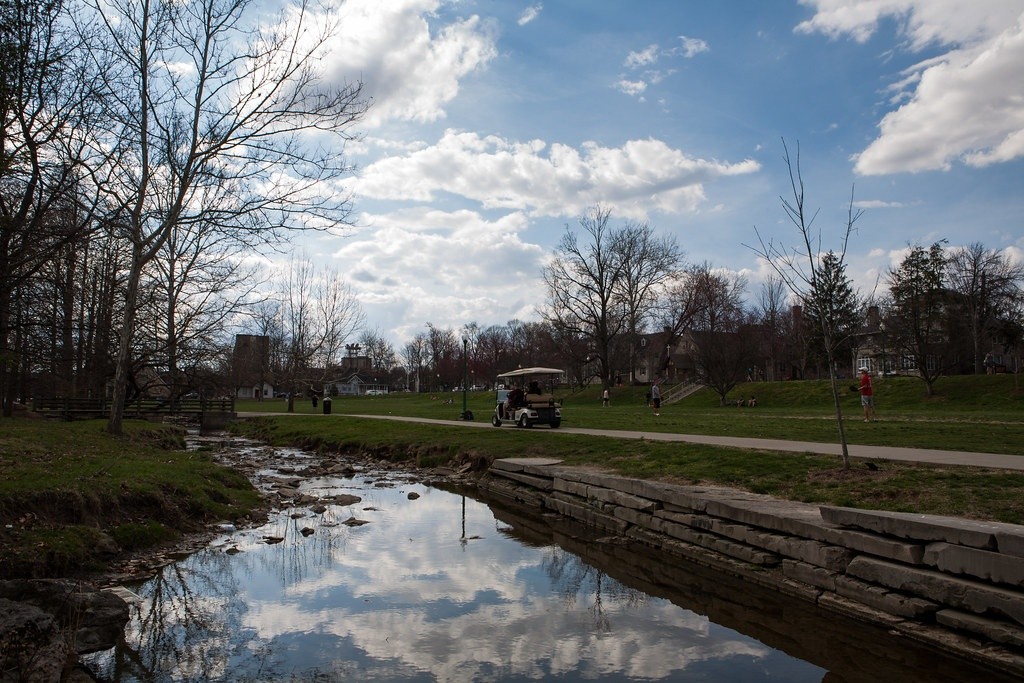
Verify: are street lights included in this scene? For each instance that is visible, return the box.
[459,333,470,422]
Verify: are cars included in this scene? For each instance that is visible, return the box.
[451,383,505,392]
[186,392,199,399]
[364,389,383,397]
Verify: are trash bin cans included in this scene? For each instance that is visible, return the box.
[323,400,331,414]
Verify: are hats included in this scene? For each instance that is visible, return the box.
[859,366,869,371]
[655,380,660,383]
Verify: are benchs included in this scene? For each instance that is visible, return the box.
[526,393,553,402]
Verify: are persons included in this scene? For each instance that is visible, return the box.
[758,365,765,383]
[746,366,754,382]
[602,388,612,408]
[730,395,746,408]
[646,390,652,407]
[616,373,622,392]
[747,396,758,408]
[651,378,662,416]
[857,367,879,423]
[312,394,319,414]
[983,349,996,375]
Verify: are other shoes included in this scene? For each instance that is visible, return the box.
[608,406,612,408]
[653,413,660,416]
[864,419,869,422]
[603,406,606,408]
